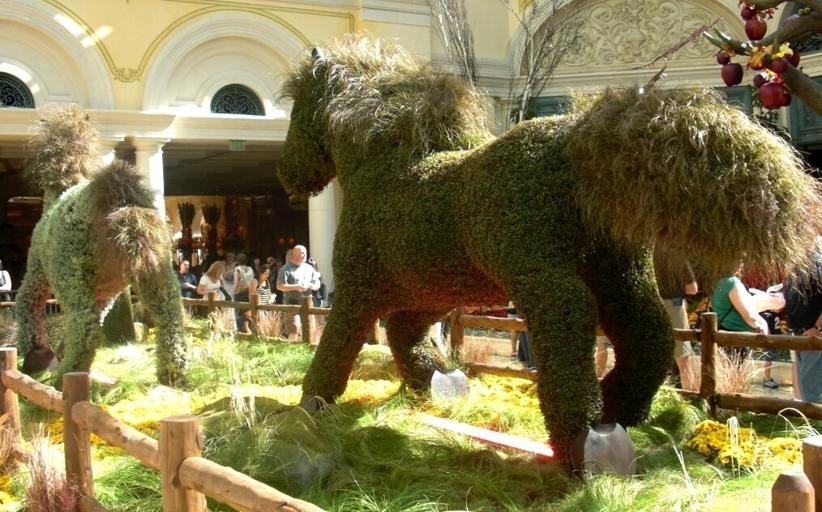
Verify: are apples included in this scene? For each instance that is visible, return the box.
[715,7,800,110]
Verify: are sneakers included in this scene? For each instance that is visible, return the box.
[762,376,778,388]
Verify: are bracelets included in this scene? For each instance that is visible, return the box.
[814,324,822,332]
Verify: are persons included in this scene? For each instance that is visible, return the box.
[782,219,822,407]
[506,300,522,356]
[788,328,803,398]
[710,256,780,389]
[652,236,699,393]
[595,334,609,381]
[170,244,327,346]
